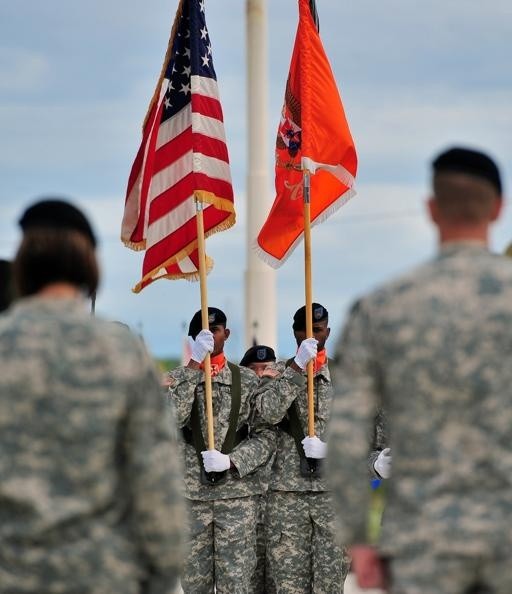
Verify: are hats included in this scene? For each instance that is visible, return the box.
[292,302,329,330]
[239,346,276,367]
[433,147,502,195]
[19,200,96,247]
[188,307,227,336]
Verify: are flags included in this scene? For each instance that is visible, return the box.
[253,1,358,267]
[120,0,237,294]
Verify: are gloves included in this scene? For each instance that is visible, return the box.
[293,338,319,370]
[301,435,327,459]
[373,448,392,480]
[190,330,215,364]
[200,450,232,473]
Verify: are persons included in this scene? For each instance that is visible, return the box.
[1,201,194,590]
[168,303,395,593]
[323,149,511,594]
